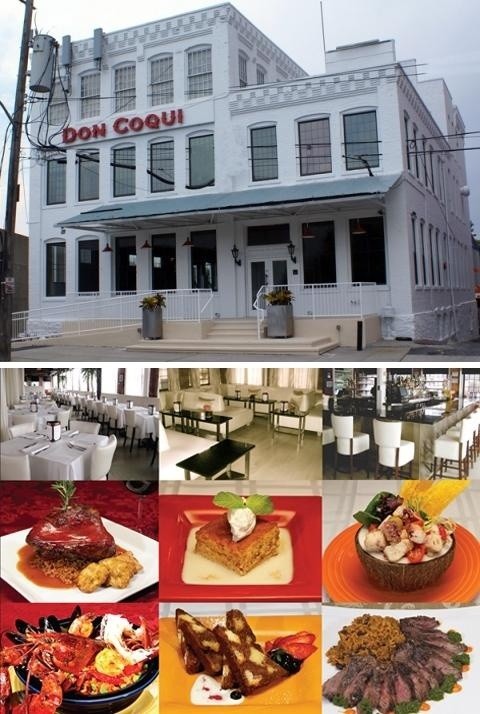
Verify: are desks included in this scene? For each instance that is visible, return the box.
[160,401,232,442]
[174,438,255,480]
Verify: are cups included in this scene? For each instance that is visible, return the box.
[200,411,212,420]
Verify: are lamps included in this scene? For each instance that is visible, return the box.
[138,222,152,249]
[287,240,297,264]
[101,225,114,252]
[231,244,242,266]
[180,221,195,248]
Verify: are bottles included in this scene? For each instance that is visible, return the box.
[290,397,296,414]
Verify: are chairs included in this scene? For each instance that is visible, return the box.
[322,392,480,479]
[1,380,158,480]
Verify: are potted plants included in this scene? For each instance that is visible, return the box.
[260,287,298,338]
[138,293,166,340]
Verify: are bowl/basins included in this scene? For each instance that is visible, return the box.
[16,618,159,712]
[356,523,456,592]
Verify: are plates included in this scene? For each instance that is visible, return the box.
[322,518,480,608]
[159,614,322,714]
[1,516,158,603]
[159,494,321,600]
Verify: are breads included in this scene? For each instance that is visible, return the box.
[175,513,293,698]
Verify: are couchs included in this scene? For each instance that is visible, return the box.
[158,381,322,439]
